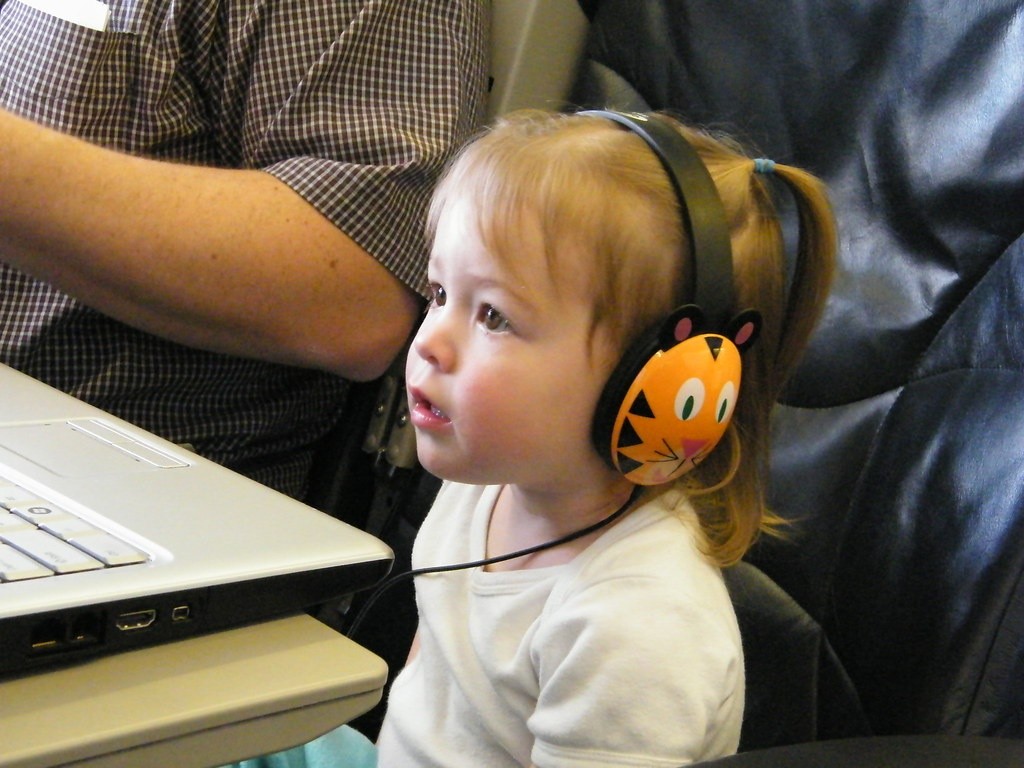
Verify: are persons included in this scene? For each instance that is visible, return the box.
[0,0,493,502]
[220,108,841,768]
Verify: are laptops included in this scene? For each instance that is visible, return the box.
[0,361,396,683]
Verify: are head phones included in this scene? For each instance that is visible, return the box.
[577,107,762,486]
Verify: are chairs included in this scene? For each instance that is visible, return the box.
[320,0,599,627]
[335,0,1023,768]
